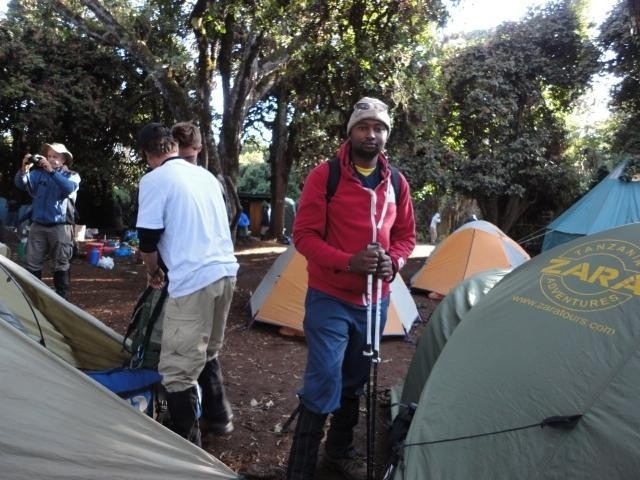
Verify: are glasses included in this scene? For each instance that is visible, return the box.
[351,101,389,113]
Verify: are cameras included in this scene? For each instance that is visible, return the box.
[29,157,42,166]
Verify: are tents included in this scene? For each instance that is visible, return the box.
[243,241,424,347]
[0,253,249,480]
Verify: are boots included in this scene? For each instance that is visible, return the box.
[31,268,42,283]
[52,270,70,301]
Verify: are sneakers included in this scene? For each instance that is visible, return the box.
[196,417,238,435]
[323,444,380,480]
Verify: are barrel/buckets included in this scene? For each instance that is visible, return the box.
[89,248,100,266]
[86,242,104,260]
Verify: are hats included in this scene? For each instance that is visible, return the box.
[136,121,174,152]
[38,141,74,167]
[344,96,393,138]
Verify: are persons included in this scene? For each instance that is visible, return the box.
[169,121,205,166]
[286,95,417,478]
[135,121,236,450]
[13,141,83,301]
